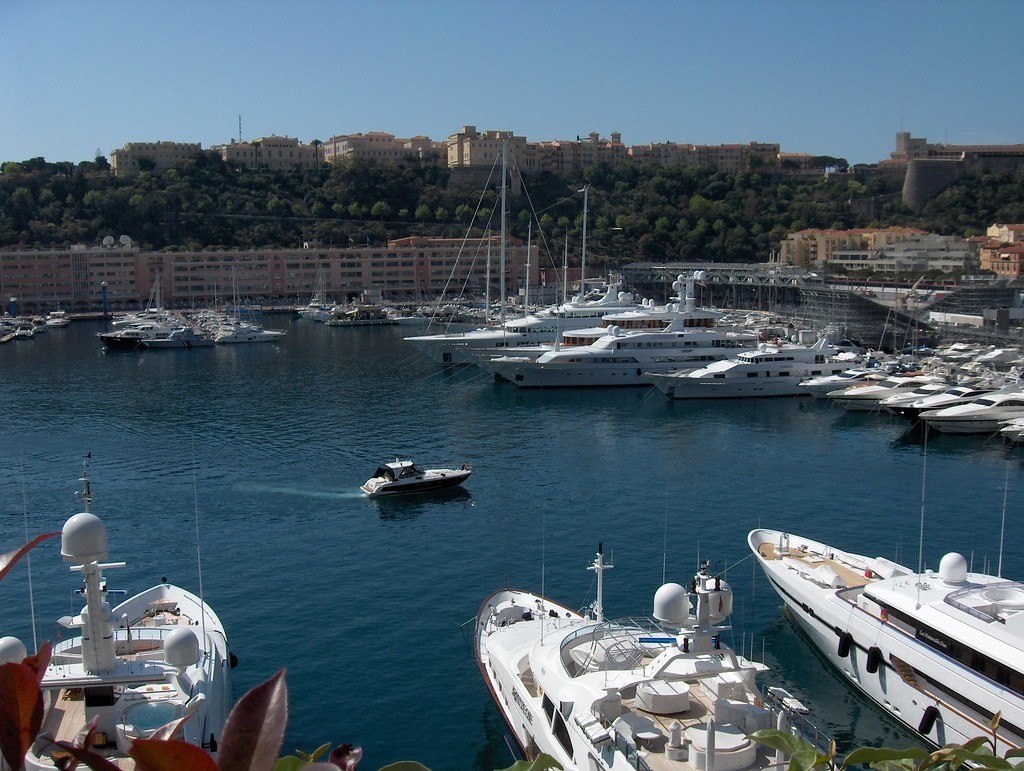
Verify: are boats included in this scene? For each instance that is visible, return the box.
[470,480,839,771]
[0,124,1022,455]
[0,443,236,771]
[746,413,1024,771]
[360,459,472,502]
